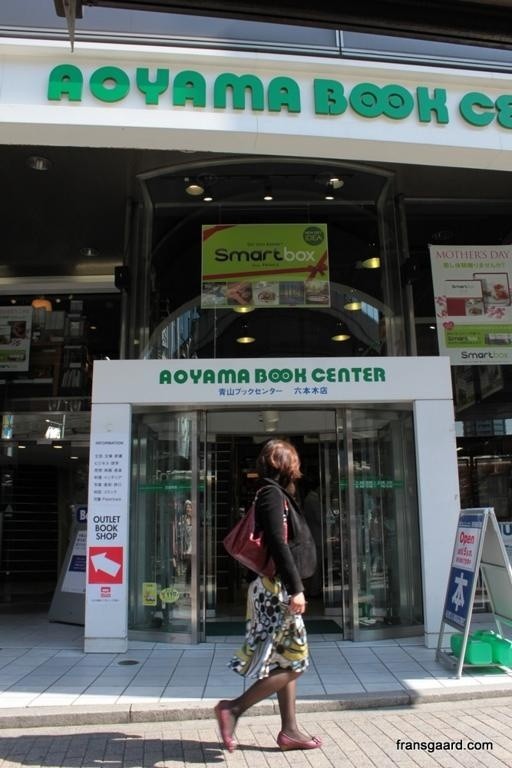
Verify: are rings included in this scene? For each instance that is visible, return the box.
[296,609,301,612]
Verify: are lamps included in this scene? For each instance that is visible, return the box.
[26,146,54,173]
[177,162,382,346]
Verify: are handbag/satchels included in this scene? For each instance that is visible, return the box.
[222,509,288,578]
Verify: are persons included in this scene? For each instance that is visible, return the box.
[172,499,195,598]
[214,439,325,754]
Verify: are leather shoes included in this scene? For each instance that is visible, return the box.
[277,731,322,751]
[215,700,236,754]
